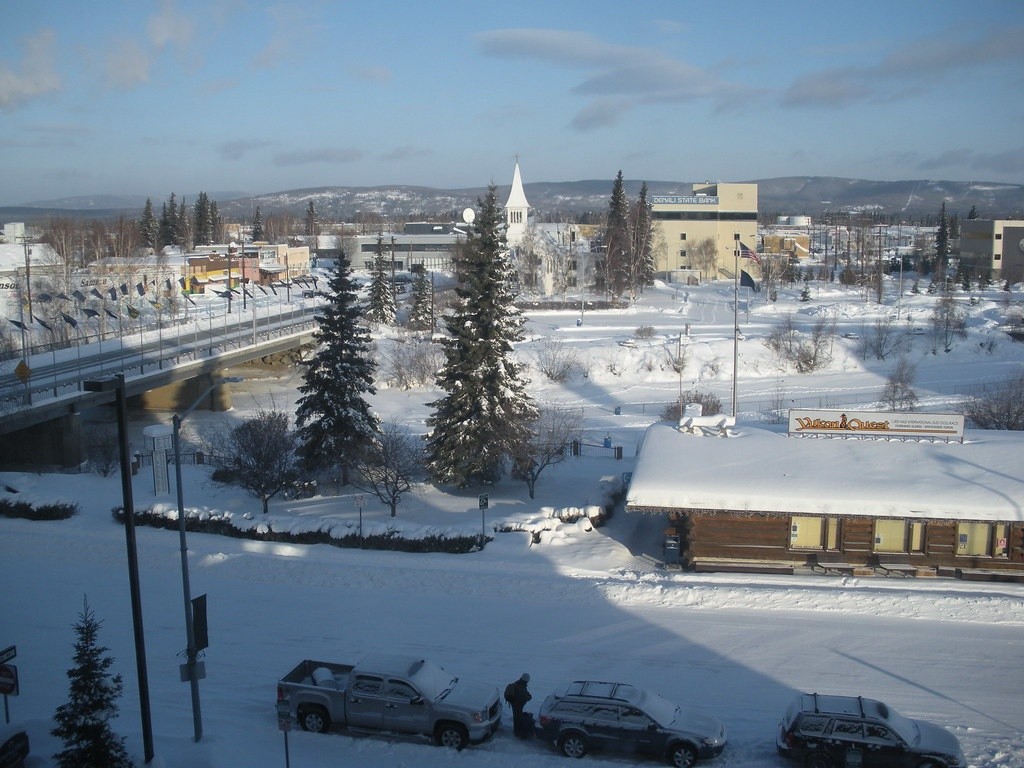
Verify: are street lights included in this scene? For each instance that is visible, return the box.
[579,245,610,321]
[171,378,250,743]
[80,370,156,764]
[897,246,924,323]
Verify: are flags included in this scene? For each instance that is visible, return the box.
[18,275,319,320]
[740,243,762,265]
[35,317,51,330]
[8,319,29,330]
[62,312,77,327]
[741,271,756,292]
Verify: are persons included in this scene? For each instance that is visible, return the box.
[512,673,532,735]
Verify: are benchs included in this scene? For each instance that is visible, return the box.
[815,552,855,576]
[878,555,918,578]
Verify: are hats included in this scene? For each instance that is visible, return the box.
[520,672,530,683]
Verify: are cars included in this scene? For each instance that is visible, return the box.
[385,273,414,284]
[293,274,318,283]
[271,277,294,288]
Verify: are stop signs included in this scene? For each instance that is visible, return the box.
[0,665,20,697]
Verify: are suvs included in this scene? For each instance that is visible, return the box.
[775,690,968,768]
[532,681,729,768]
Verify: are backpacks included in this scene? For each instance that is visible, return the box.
[503,680,525,706]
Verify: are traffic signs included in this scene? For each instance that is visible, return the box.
[0,644,16,664]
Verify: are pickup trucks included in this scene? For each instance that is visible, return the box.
[275,651,503,753]
